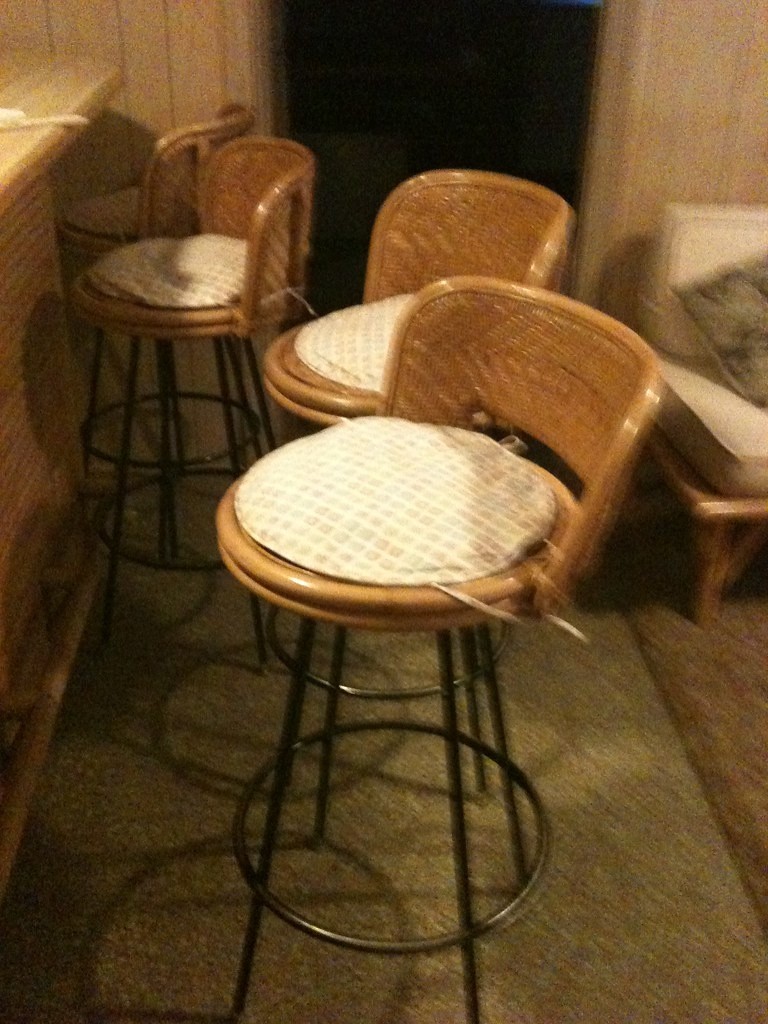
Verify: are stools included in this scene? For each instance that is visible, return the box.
[71,134,314,655]
[210,275,666,1024]
[261,169,574,834]
[65,100,254,560]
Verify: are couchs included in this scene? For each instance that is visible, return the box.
[635,204,768,629]
